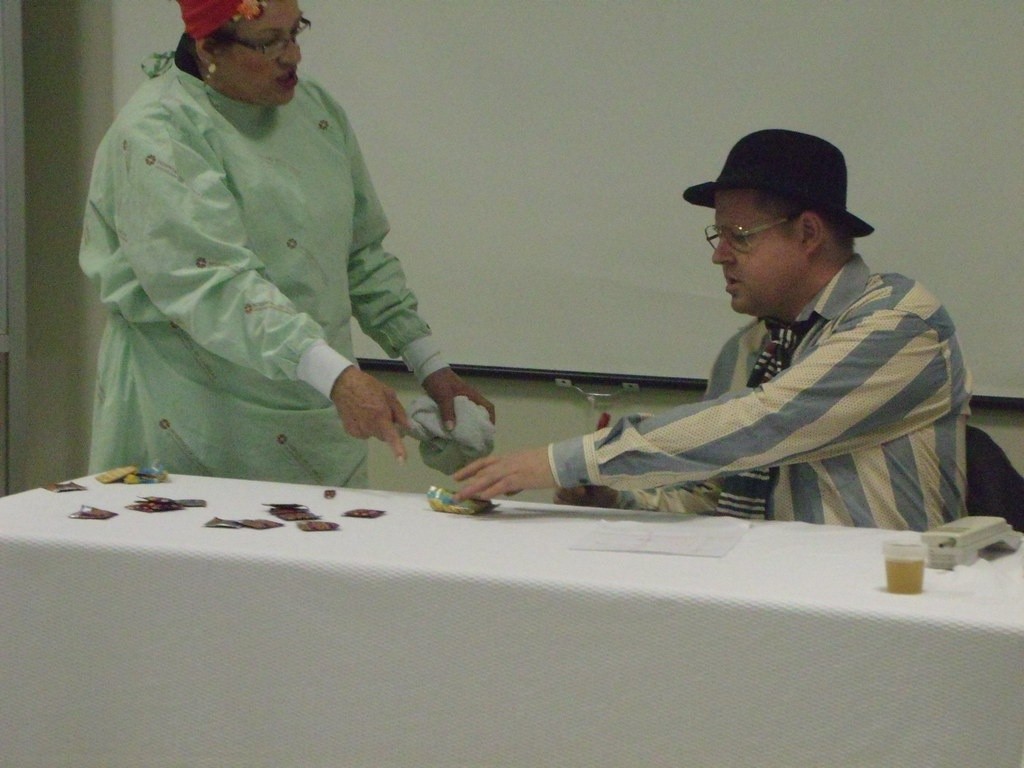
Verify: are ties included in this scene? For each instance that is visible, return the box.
[715,311,820,520]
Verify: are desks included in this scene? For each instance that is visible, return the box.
[0,472,1024,768]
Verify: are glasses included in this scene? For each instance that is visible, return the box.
[218,16,311,68]
[705,209,803,252]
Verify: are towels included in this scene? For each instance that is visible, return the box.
[395,395,498,459]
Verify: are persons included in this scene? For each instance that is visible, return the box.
[79,0,496,487]
[453,129,969,532]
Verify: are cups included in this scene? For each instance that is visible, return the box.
[883,539,927,594]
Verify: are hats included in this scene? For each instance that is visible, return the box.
[176,0,267,38]
[684,129,874,237]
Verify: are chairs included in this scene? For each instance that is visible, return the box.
[964,424,1024,536]
